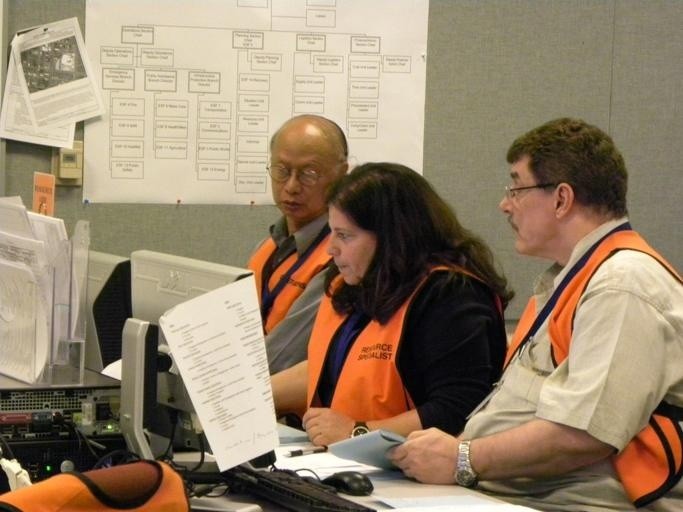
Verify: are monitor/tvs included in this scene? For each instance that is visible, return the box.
[120,248,253,489]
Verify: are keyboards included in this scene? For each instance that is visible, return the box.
[235,472,374,512]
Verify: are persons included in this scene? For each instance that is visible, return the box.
[246,115,350,424]
[270,163,511,450]
[386,118,683,512]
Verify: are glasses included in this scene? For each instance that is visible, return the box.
[507,182,557,200]
[265,157,346,185]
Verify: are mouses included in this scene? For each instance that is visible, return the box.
[325,470,372,495]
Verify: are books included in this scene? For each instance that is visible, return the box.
[327,430,408,471]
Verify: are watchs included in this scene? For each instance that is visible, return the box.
[454,443,478,488]
[352,420,370,439]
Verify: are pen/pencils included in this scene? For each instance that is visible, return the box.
[282,445,328,457]
